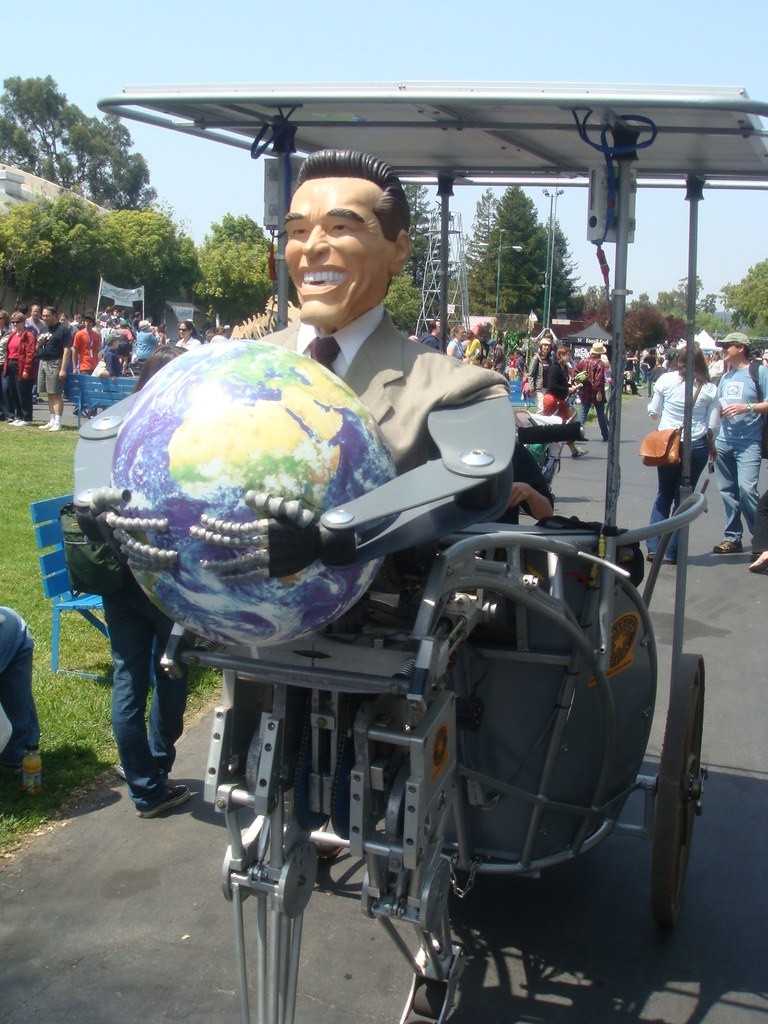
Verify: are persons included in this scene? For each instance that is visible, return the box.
[0,304,232,432]
[103,345,190,816]
[646,345,720,564]
[713,333,768,560]
[0,606,40,773]
[501,444,554,524]
[625,342,768,397]
[73,148,518,578]
[408,321,610,459]
[749,490,768,573]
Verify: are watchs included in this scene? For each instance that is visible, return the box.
[746,403,751,412]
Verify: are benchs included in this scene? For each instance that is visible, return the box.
[62,372,139,429]
[28,493,112,682]
[509,380,537,407]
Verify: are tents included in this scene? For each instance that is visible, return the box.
[661,330,722,351]
[567,322,613,366]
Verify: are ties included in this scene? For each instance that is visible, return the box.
[310,337,340,373]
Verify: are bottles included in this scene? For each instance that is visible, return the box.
[22,750,42,798]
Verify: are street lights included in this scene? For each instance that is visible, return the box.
[495,228,523,314]
[543,188,565,329]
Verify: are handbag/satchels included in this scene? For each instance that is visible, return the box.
[575,371,589,382]
[59,513,124,595]
[639,429,682,466]
[521,376,533,397]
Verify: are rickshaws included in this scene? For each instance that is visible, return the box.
[70,81,767,1024]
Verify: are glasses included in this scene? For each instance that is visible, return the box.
[722,344,739,349]
[179,329,188,332]
[11,321,23,324]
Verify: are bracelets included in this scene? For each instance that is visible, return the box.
[750,403,755,414]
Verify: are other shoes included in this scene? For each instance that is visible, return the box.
[647,554,655,560]
[136,785,190,817]
[662,559,676,565]
[578,437,589,441]
[0,744,32,773]
[5,416,62,431]
[116,763,127,779]
[571,449,588,458]
[749,551,768,570]
[713,541,743,553]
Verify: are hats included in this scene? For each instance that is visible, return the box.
[541,338,552,344]
[486,339,496,345]
[715,332,750,347]
[590,342,606,354]
[81,317,154,333]
